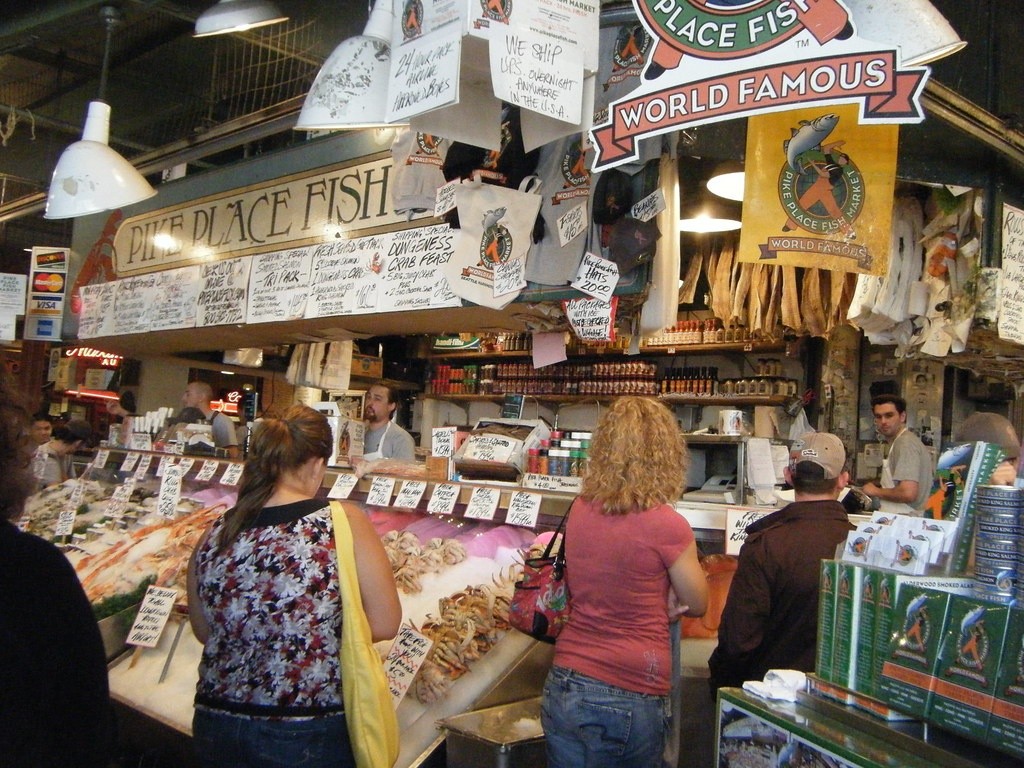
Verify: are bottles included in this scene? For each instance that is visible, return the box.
[528,431,592,478]
[638,317,752,347]
[430,356,797,398]
[497,332,532,352]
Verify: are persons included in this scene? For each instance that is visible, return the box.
[106,383,238,457]
[31,420,91,493]
[706,432,857,703]
[31,413,53,444]
[236,395,263,447]
[363,383,415,461]
[861,394,931,516]
[187,405,402,768]
[541,396,709,768]
[0,386,109,768]
[954,411,1020,486]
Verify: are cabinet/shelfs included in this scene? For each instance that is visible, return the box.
[424,340,804,407]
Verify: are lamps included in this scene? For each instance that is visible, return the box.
[43,6,158,220]
[294,0,413,130]
[191,0,292,38]
[679,158,745,234]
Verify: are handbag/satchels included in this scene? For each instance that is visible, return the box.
[509,495,578,643]
[330,499,399,768]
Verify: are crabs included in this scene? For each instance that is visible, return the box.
[474,542,559,632]
[409,614,479,681]
[381,531,466,592]
[406,660,446,704]
[437,591,497,652]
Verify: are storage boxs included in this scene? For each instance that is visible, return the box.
[350,352,382,378]
[107,414,215,455]
[817,440,1024,759]
[453,418,552,473]
[325,415,366,469]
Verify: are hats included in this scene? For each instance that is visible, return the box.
[167,408,203,428]
[789,433,845,482]
[65,420,94,448]
[957,414,1020,464]
